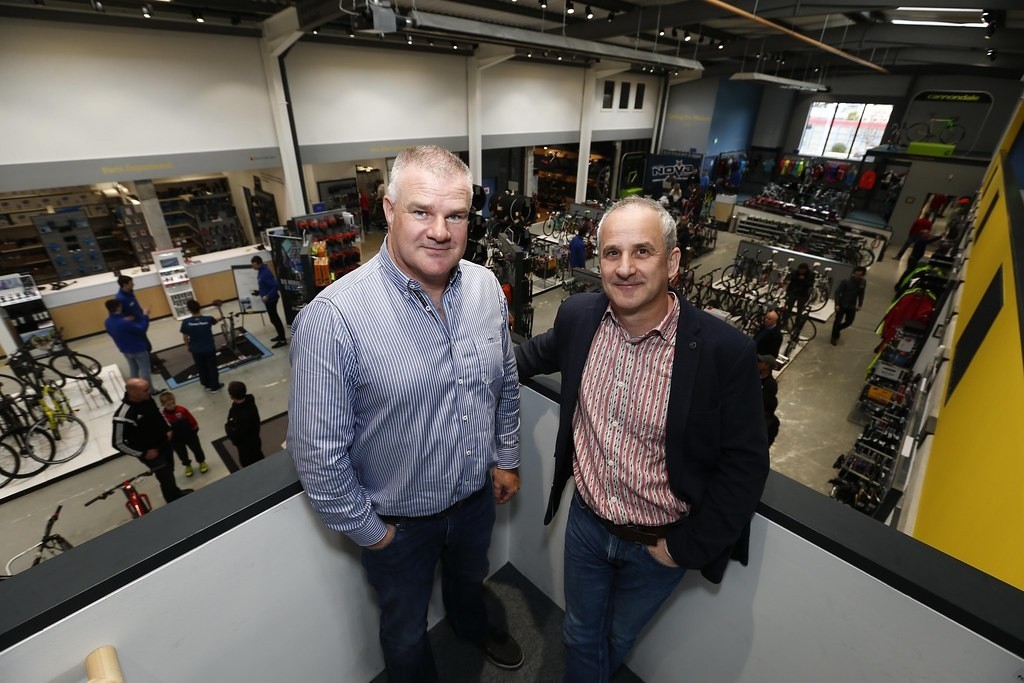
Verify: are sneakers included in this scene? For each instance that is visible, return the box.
[453,622,524,670]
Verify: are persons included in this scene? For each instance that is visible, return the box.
[752,309,784,360]
[894,211,951,260]
[179,300,226,394]
[675,214,691,268]
[782,263,816,330]
[700,168,709,186]
[829,265,867,347]
[681,182,704,222]
[251,256,286,349]
[511,194,781,683]
[568,227,587,277]
[112,377,194,504]
[289,145,523,683]
[160,392,209,478]
[105,276,162,395]
[224,381,265,467]
[756,353,780,448]
[667,181,684,212]
[894,226,935,290]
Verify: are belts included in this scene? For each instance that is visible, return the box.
[590,509,690,546]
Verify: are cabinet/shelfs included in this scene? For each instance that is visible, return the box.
[157,196,202,248]
[0,235,119,269]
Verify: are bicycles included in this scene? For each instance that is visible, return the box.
[484,174,878,376]
[5,503,76,579]
[0,325,103,491]
[82,471,158,522]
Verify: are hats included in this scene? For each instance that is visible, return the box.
[757,354,778,370]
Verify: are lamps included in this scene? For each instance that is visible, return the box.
[31,0,204,24]
[312,27,481,51]
[527,0,725,77]
[980,9,998,61]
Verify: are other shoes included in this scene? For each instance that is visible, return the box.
[152,389,166,397]
[271,336,280,341]
[199,462,207,473]
[211,383,225,394]
[272,340,287,348]
[205,385,211,391]
[181,489,193,496]
[186,465,193,477]
[831,338,836,345]
